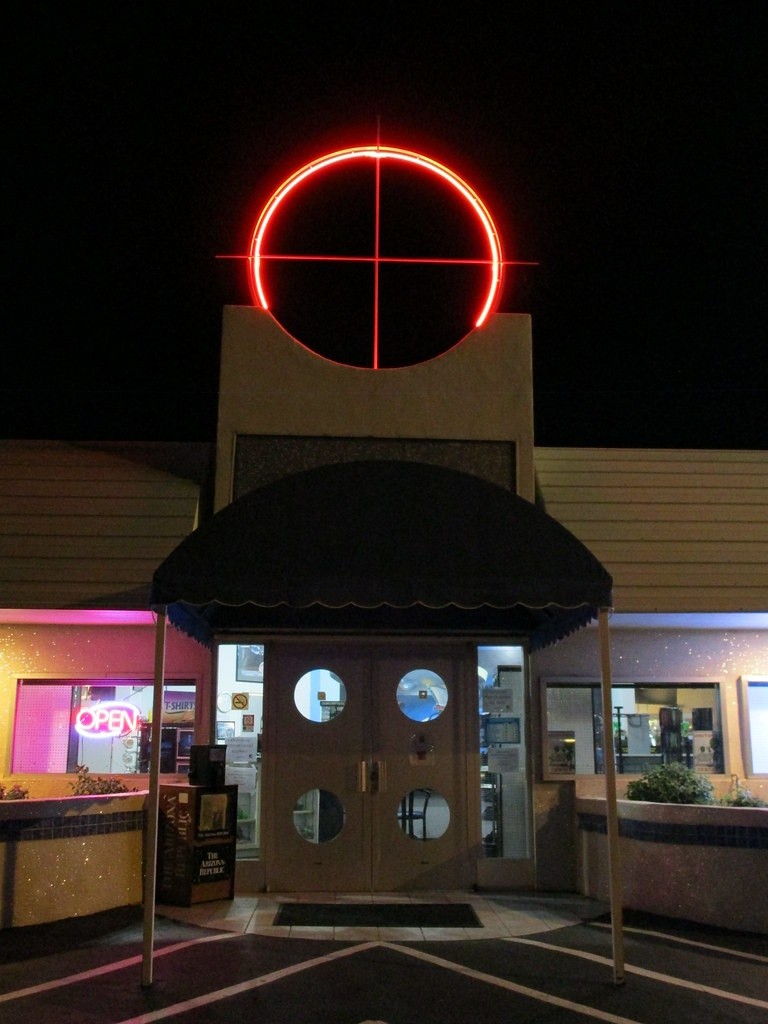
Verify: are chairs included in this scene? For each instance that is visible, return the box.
[397,788,432,841]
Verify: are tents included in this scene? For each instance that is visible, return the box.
[140,458,624,984]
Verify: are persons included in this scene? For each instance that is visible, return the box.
[656,708,684,770]
